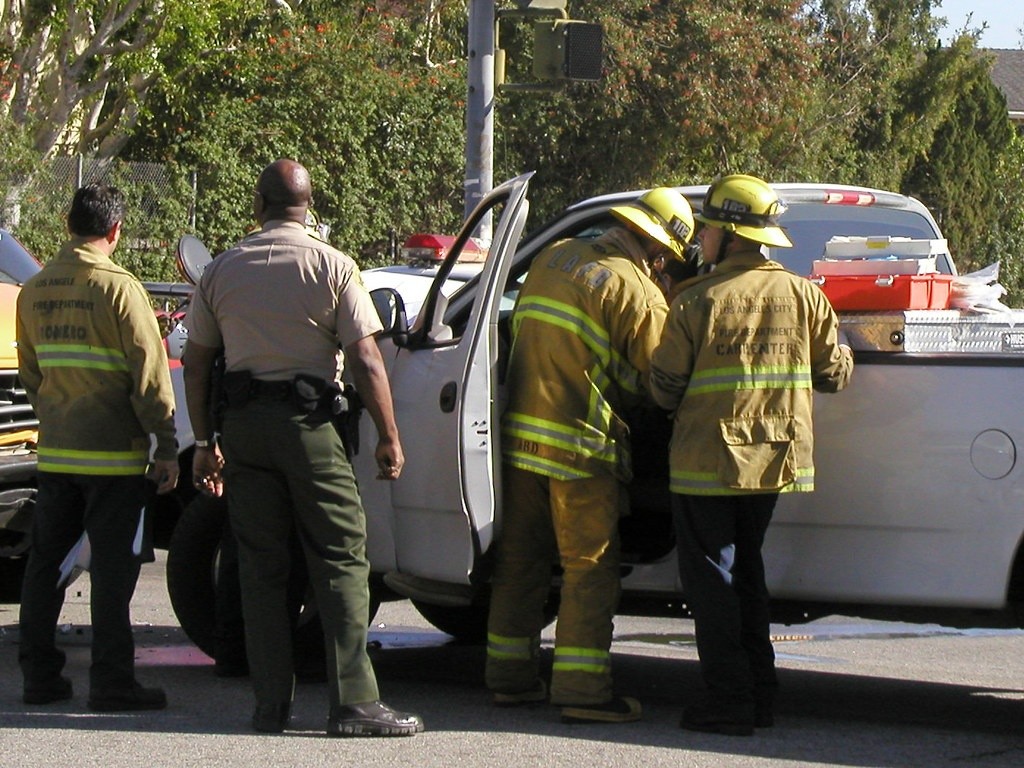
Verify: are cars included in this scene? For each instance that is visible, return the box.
[139,280,222,547]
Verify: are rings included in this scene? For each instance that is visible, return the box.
[201,478,208,484]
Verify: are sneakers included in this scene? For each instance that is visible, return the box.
[252,702,293,733]
[23,677,73,705]
[327,699,424,736]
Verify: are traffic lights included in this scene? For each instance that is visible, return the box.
[531,18,605,84]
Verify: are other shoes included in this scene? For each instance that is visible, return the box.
[562,697,642,725]
[493,683,546,707]
[754,706,774,727]
[88,687,167,712]
[679,705,754,736]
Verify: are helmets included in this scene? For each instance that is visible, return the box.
[608,187,694,263]
[692,174,793,248]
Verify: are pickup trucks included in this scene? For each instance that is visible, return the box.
[167,171,1023,682]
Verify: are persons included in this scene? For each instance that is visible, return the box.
[484,188,696,724]
[183,160,425,737]
[16,182,180,712]
[648,173,854,736]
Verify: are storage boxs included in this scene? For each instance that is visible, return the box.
[809,273,956,312]
[836,309,1024,354]
[813,238,948,274]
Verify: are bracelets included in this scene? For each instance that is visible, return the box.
[195,436,215,447]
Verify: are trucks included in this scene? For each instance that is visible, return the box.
[0,229,86,597]
